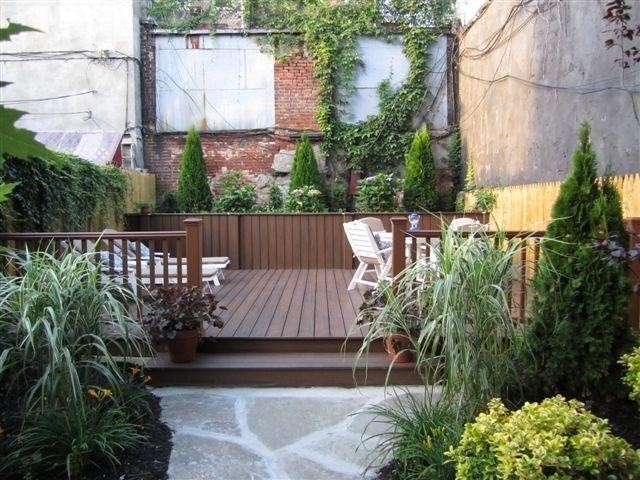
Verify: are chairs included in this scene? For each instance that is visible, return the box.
[343,214,486,302]
[60,227,230,298]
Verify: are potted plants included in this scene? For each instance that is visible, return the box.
[355,283,427,361]
[139,281,228,366]
